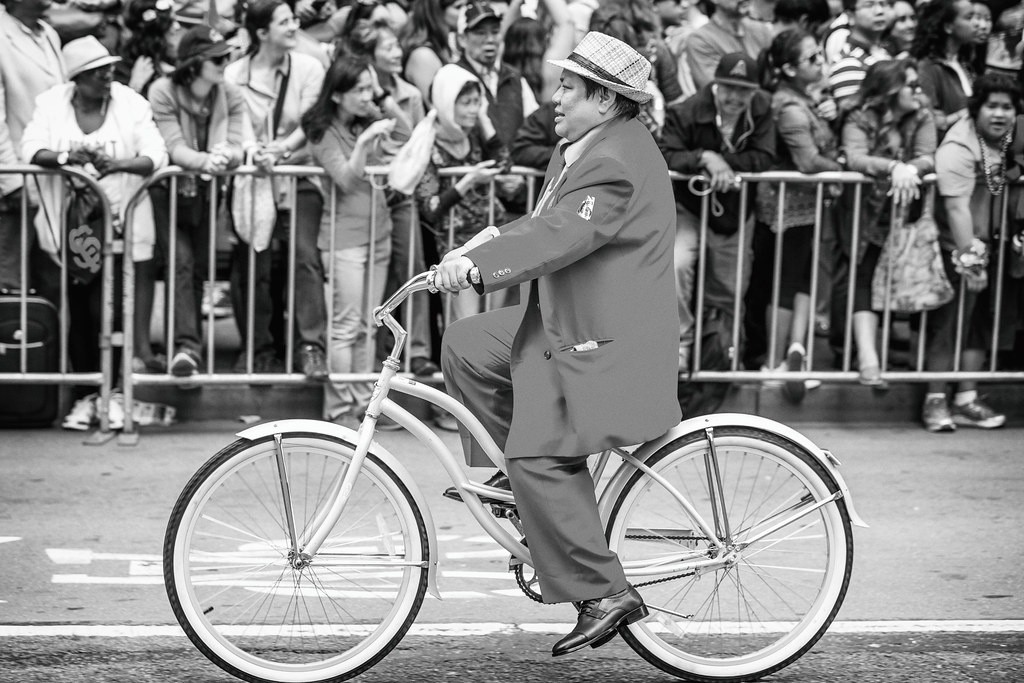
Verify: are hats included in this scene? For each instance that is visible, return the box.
[457,2,499,35]
[546,31,655,105]
[713,52,763,89]
[62,34,124,79]
[167,27,242,77]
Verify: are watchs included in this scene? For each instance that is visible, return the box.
[283,145,292,160]
[57,150,71,165]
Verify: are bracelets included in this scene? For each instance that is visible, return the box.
[372,89,392,106]
[888,160,901,174]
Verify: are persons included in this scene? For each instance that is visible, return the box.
[0,0,1023,433]
[434,32,681,658]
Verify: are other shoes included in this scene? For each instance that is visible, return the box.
[60,338,1010,431]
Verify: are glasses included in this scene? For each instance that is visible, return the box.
[204,53,233,67]
[853,0,888,11]
[899,78,921,93]
[793,48,823,67]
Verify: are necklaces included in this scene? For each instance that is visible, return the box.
[977,131,1011,196]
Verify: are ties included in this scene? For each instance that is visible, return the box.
[549,152,566,192]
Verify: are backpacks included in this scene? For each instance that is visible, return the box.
[0,288,85,430]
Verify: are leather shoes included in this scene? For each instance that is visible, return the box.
[551,583,650,658]
[443,470,515,503]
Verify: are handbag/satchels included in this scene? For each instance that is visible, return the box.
[232,142,277,251]
[56,154,111,282]
[870,156,958,315]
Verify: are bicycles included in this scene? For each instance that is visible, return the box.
[161,264,871,683]
